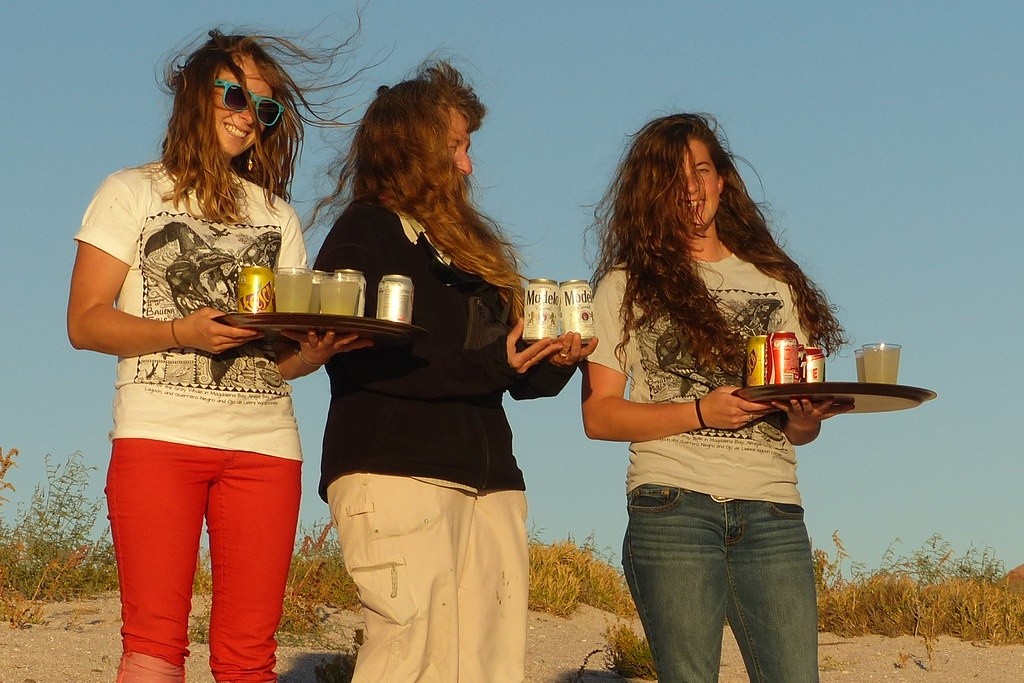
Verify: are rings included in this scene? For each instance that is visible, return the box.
[749,414,754,422]
[559,352,567,358]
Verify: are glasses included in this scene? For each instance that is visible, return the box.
[215,80,285,126]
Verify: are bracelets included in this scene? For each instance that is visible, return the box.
[170,318,185,350]
[695,397,707,430]
[297,349,327,367]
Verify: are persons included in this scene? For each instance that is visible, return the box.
[66,32,370,683]
[309,71,599,683]
[583,112,856,682]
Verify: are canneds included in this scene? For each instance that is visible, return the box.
[746,330,827,386]
[559,279,596,343]
[522,277,559,342]
[376,274,414,325]
[238,266,366,318]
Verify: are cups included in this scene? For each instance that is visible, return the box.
[854,343,902,385]
[273,266,360,316]
